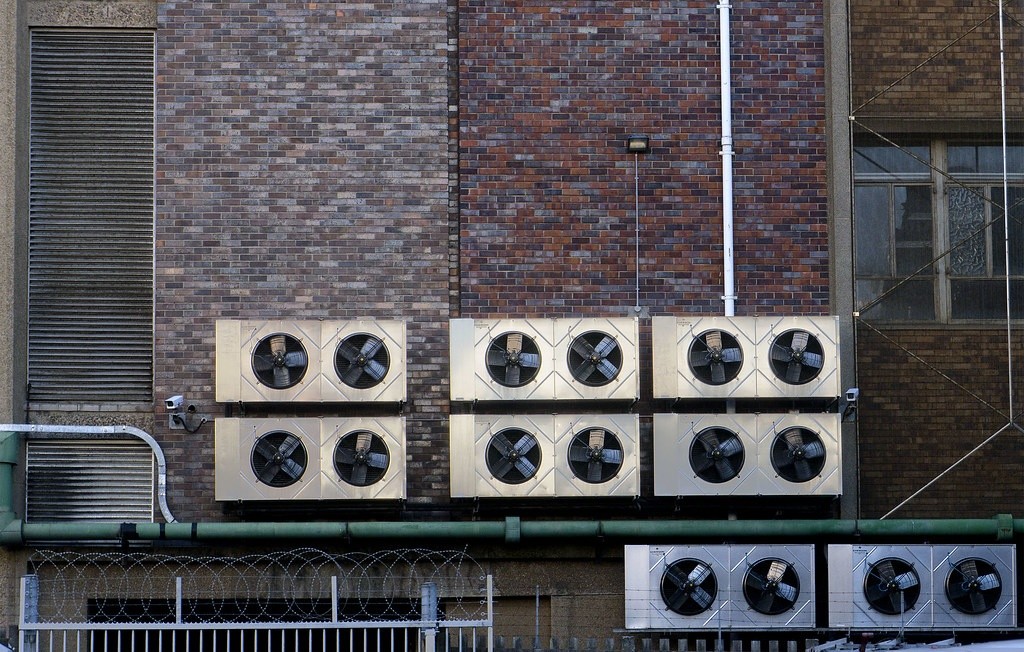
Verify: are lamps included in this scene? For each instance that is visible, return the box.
[626,134,650,153]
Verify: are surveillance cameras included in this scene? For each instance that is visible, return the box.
[164,396,184,410]
[846,388,859,402]
